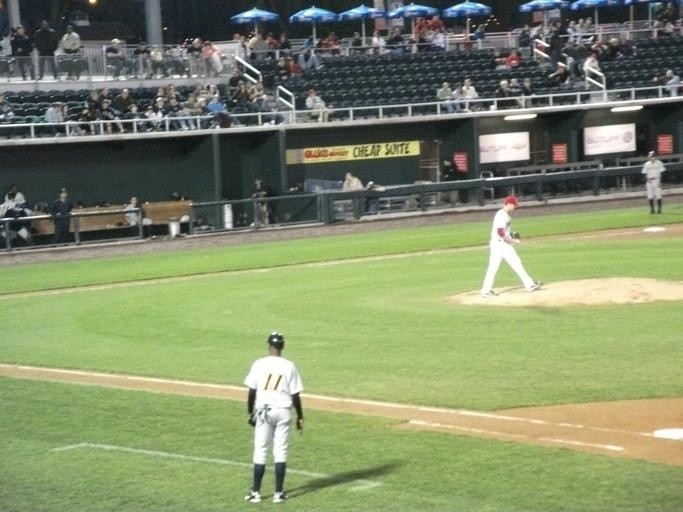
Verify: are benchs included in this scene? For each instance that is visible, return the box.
[31,198,190,237]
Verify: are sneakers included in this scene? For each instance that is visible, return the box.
[480,290,499,297]
[244,489,261,503]
[273,490,289,503]
[529,281,543,292]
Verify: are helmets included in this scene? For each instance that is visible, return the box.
[59,187,69,194]
[171,192,180,200]
[268,333,285,345]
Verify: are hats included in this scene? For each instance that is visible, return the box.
[504,197,519,208]
[649,151,656,157]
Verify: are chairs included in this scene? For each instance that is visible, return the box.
[256,50,573,119]
[575,34,682,101]
[2,85,268,129]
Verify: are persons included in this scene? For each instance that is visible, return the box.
[240,331,304,504]
[123,194,152,226]
[664,70,681,96]
[442,158,459,183]
[3,184,28,217]
[492,47,537,108]
[368,16,445,56]
[342,171,364,191]
[650,1,676,39]
[517,19,633,87]
[49,187,72,244]
[1,19,284,138]
[251,178,269,224]
[237,32,366,86]
[476,24,487,37]
[477,194,544,296]
[437,78,481,113]
[0,191,37,244]
[640,151,666,215]
[305,88,335,122]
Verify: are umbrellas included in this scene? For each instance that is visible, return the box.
[287,6,339,46]
[386,2,440,38]
[229,7,280,38]
[518,1,682,17]
[337,3,386,45]
[441,0,493,39]
